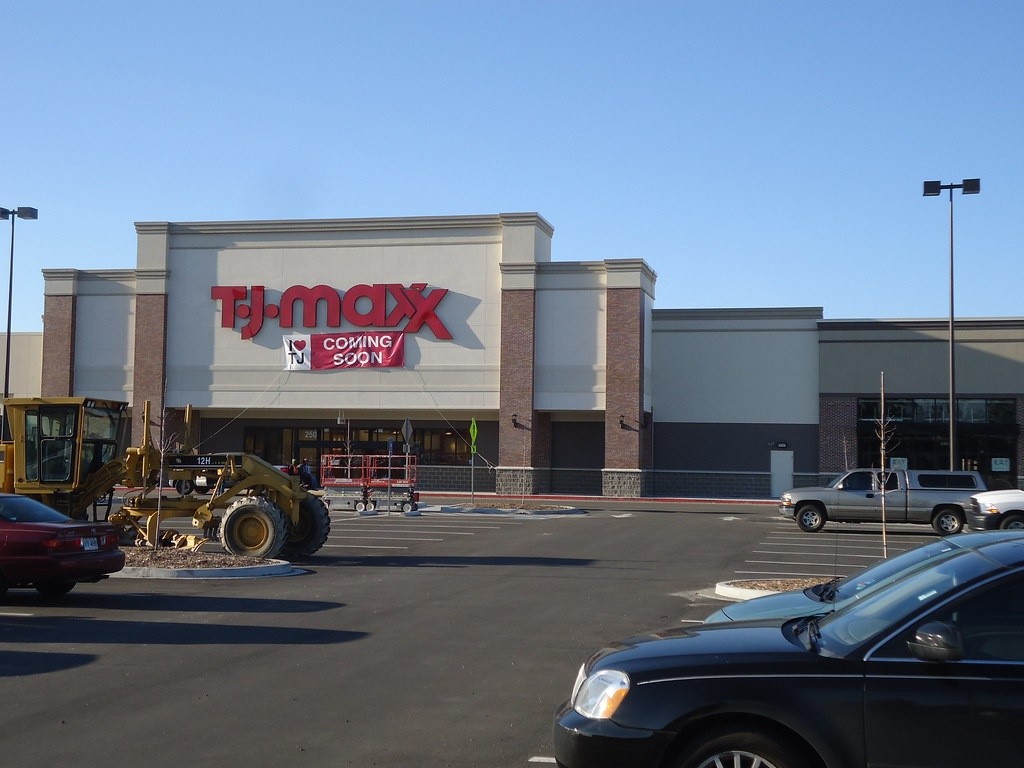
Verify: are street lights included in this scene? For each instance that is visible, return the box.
[0,207,38,437]
[922,178,979,471]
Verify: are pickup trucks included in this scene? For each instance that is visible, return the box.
[777,468,987,533]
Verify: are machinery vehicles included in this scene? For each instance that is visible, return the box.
[0,390,332,563]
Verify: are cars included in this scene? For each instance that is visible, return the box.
[964,489,1024,530]
[552,538,1024,768]
[700,524,1024,634]
[0,491,125,605]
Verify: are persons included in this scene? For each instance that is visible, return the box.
[288,458,312,490]
